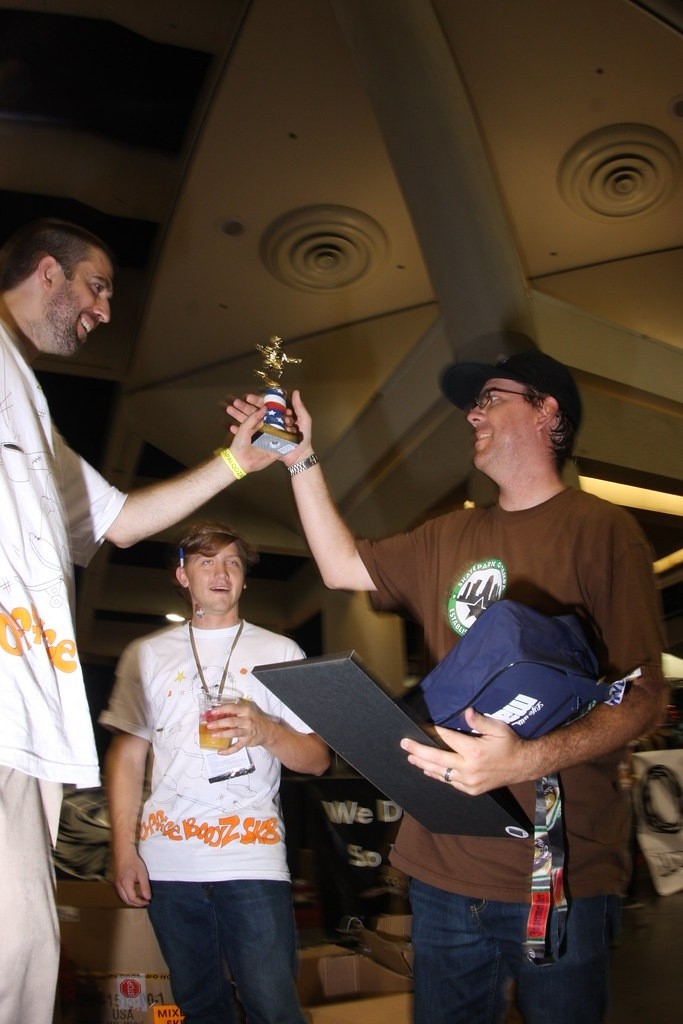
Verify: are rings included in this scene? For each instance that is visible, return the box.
[444,768,451,781]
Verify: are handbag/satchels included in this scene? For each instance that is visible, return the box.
[397,599,608,740]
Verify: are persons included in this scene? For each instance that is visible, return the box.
[227,350,669,1024]
[0,219,297,1024]
[96,522,331,1024]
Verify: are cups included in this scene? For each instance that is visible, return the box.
[195,692,241,752]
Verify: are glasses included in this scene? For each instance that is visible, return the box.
[464,386,545,421]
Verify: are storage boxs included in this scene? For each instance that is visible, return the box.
[294,913,415,1023]
[55,882,177,1024]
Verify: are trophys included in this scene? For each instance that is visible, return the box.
[252,334,303,455]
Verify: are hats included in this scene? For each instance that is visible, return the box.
[441,351,581,430]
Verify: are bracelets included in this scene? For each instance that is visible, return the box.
[221,448,246,481]
[288,454,319,476]
